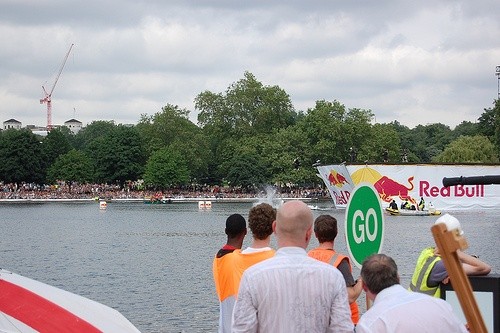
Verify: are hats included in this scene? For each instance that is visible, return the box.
[435,212,464,235]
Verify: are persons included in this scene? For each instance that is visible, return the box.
[307,215,363,333]
[0,179,331,204]
[233,202,277,302]
[409,214,492,298]
[387,196,437,213]
[231,200,355,333]
[213,213,248,333]
[356,253,470,333]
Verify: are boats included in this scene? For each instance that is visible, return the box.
[386,207,442,216]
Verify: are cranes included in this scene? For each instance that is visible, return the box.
[40,43,75,130]
